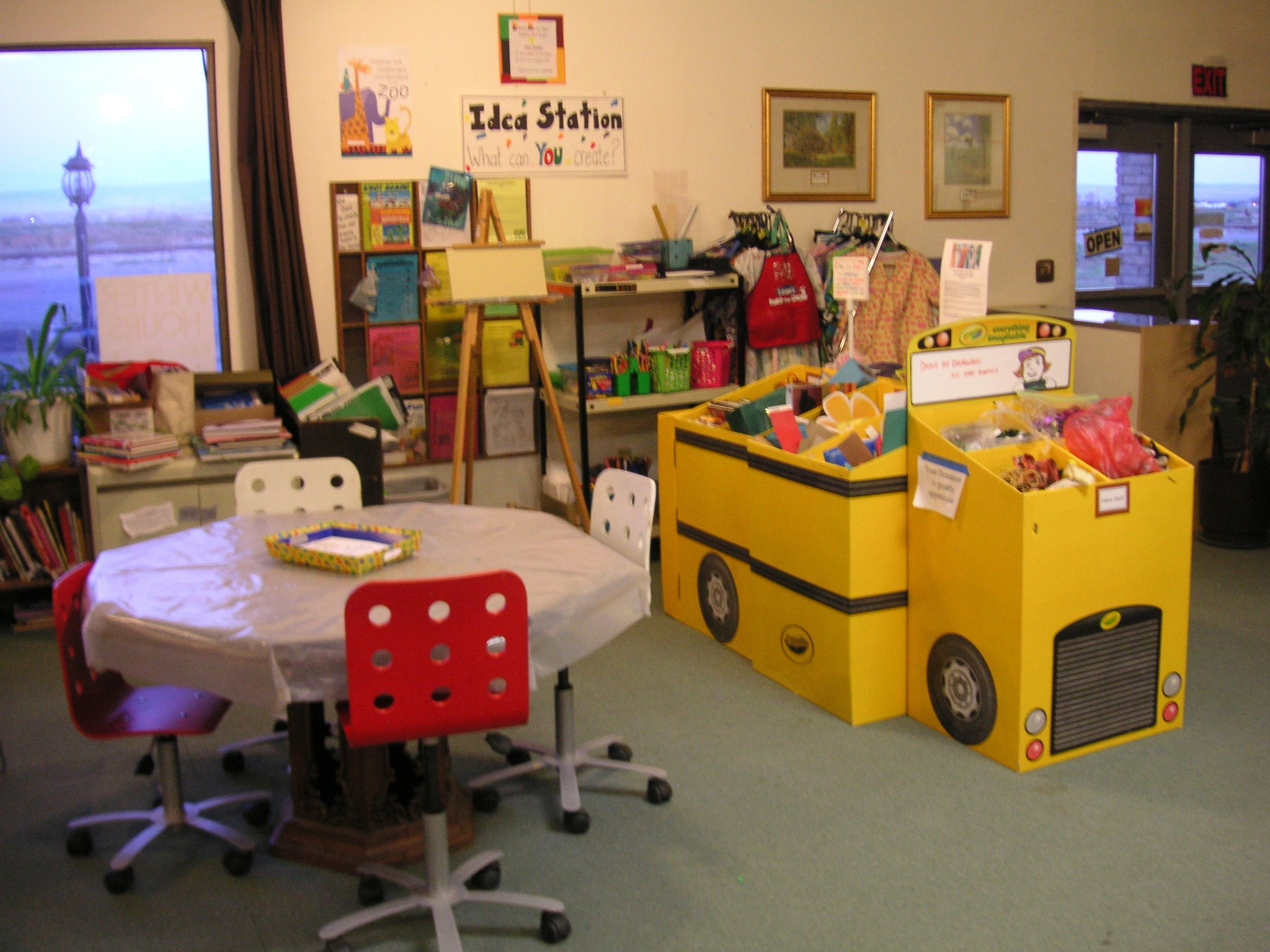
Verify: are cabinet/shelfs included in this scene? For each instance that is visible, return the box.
[86,438,303,553]
[526,272,746,520]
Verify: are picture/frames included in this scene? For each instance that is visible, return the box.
[760,85,879,204]
[924,91,1015,221]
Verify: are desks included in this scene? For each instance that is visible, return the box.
[77,503,654,881]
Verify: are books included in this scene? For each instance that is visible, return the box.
[1,350,412,642]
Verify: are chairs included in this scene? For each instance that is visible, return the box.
[463,462,673,836]
[45,561,276,893]
[212,461,422,806]
[314,568,575,952]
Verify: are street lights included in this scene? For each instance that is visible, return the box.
[61,142,100,366]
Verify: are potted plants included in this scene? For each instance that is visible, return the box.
[1176,240,1268,549]
[0,306,109,505]
